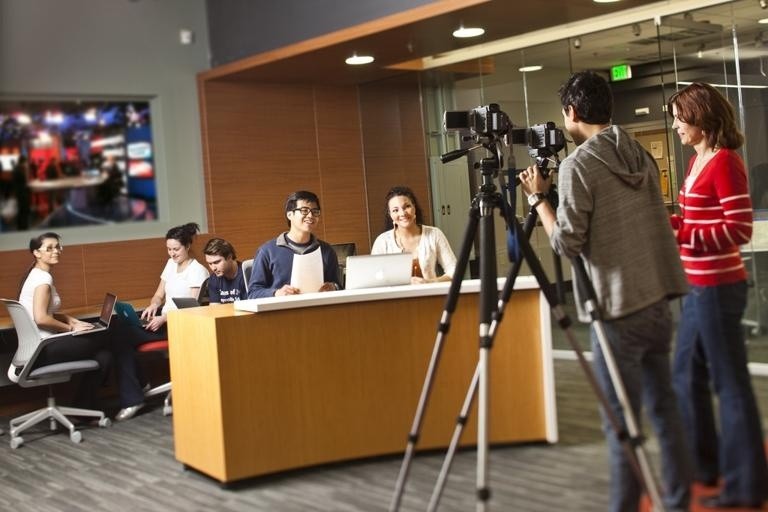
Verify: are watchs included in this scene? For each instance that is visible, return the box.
[526,191,549,208]
[333,282,340,291]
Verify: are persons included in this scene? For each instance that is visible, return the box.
[664,76,766,512]
[202,238,250,308]
[517,70,691,512]
[248,191,345,301]
[12,150,121,230]
[114,222,210,425]
[15,233,111,422]
[366,185,464,285]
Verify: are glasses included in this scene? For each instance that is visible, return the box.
[38,246,63,253]
[294,207,320,216]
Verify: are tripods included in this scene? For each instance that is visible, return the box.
[426,159,668,512]
[390,136,668,512]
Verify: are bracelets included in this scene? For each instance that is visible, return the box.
[68,325,72,331]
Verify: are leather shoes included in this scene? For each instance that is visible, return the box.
[700,490,734,506]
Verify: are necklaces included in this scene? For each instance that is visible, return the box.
[695,151,707,172]
[398,226,419,248]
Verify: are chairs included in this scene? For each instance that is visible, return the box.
[0,298,110,449]
[330,243,357,289]
[241,259,254,292]
[135,339,172,416]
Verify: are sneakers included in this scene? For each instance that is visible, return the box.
[116,402,145,420]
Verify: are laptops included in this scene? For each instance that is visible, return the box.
[172,297,200,309]
[345,252,414,290]
[114,301,151,328]
[71,292,118,336]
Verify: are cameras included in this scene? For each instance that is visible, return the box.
[443,102,514,136]
[504,121,567,159]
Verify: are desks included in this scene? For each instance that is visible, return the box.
[165,276,559,489]
